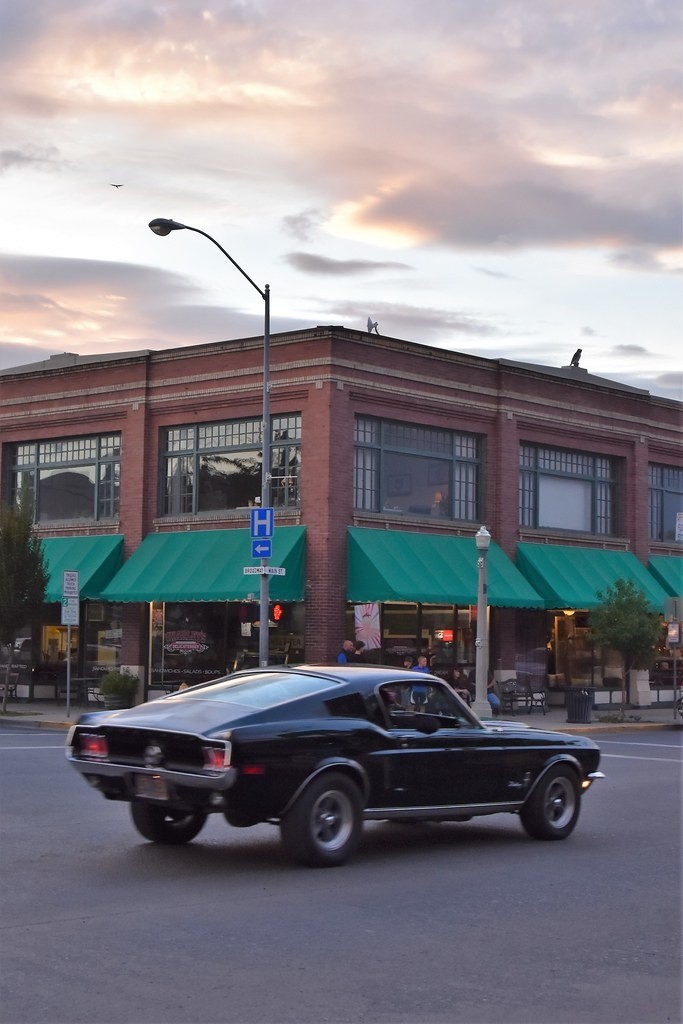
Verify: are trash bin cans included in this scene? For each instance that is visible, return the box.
[561,685,597,723]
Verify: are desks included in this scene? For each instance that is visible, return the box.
[71,677,105,708]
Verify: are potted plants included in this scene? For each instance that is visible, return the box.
[99,670,140,709]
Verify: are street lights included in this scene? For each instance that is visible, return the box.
[149,218,271,668]
[469,525,494,720]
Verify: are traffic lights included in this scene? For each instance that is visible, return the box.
[270,601,291,626]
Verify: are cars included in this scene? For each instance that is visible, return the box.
[65,662,607,867]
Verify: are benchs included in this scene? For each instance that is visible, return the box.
[497,679,547,716]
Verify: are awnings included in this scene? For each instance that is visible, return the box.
[346,523,547,610]
[99,525,308,603]
[647,555,683,598]
[516,541,669,614]
[30,534,126,603]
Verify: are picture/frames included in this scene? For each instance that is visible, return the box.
[386,472,412,497]
[427,461,449,485]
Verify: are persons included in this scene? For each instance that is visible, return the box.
[353,640,368,663]
[336,640,353,664]
[486,694,501,718]
[402,656,413,707]
[446,666,471,703]
[410,656,429,714]
